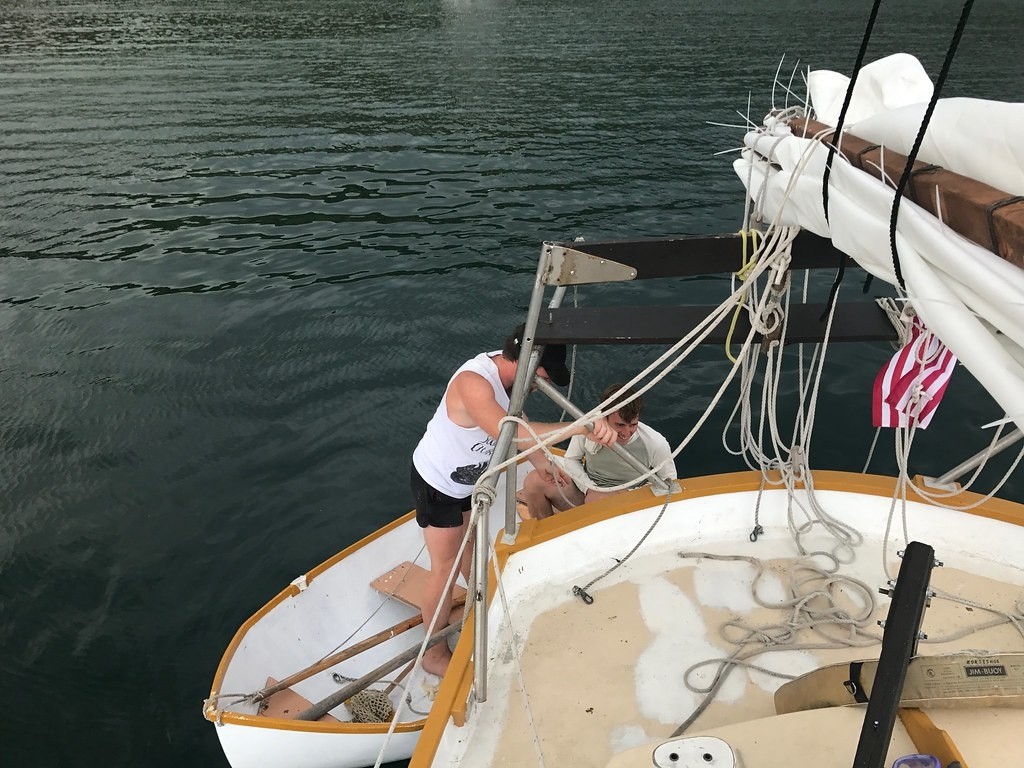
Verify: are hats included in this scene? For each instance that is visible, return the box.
[515,322,572,387]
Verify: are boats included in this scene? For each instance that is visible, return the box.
[202,0,1024,768]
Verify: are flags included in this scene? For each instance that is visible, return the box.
[870,300,959,430]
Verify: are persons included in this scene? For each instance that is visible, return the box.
[411,324,572,680]
[524,383,678,520]
